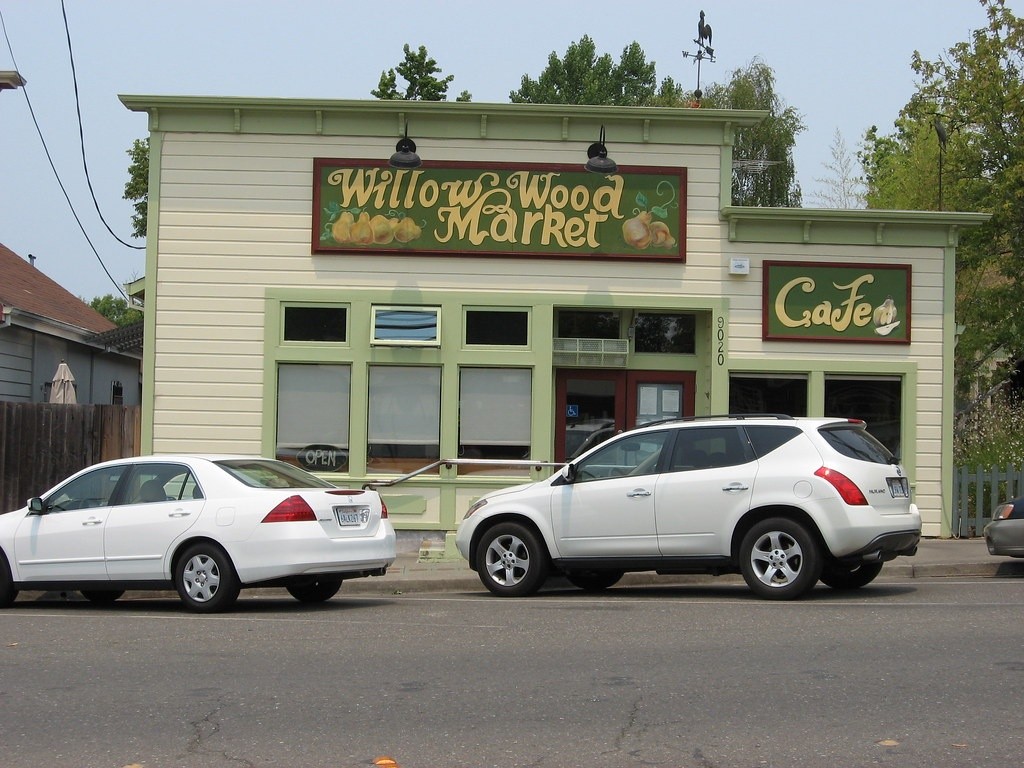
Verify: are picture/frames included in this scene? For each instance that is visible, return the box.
[762,260,912,344]
[312,157,688,263]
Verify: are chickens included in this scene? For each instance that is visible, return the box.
[698,10,712,46]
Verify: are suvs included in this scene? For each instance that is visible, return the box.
[455,413,921,601]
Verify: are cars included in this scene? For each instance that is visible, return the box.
[0,454,397,612]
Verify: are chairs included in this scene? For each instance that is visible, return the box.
[688,450,727,468]
[138,480,166,503]
[192,484,203,499]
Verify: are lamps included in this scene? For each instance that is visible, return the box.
[585,124,618,174]
[388,118,423,169]
